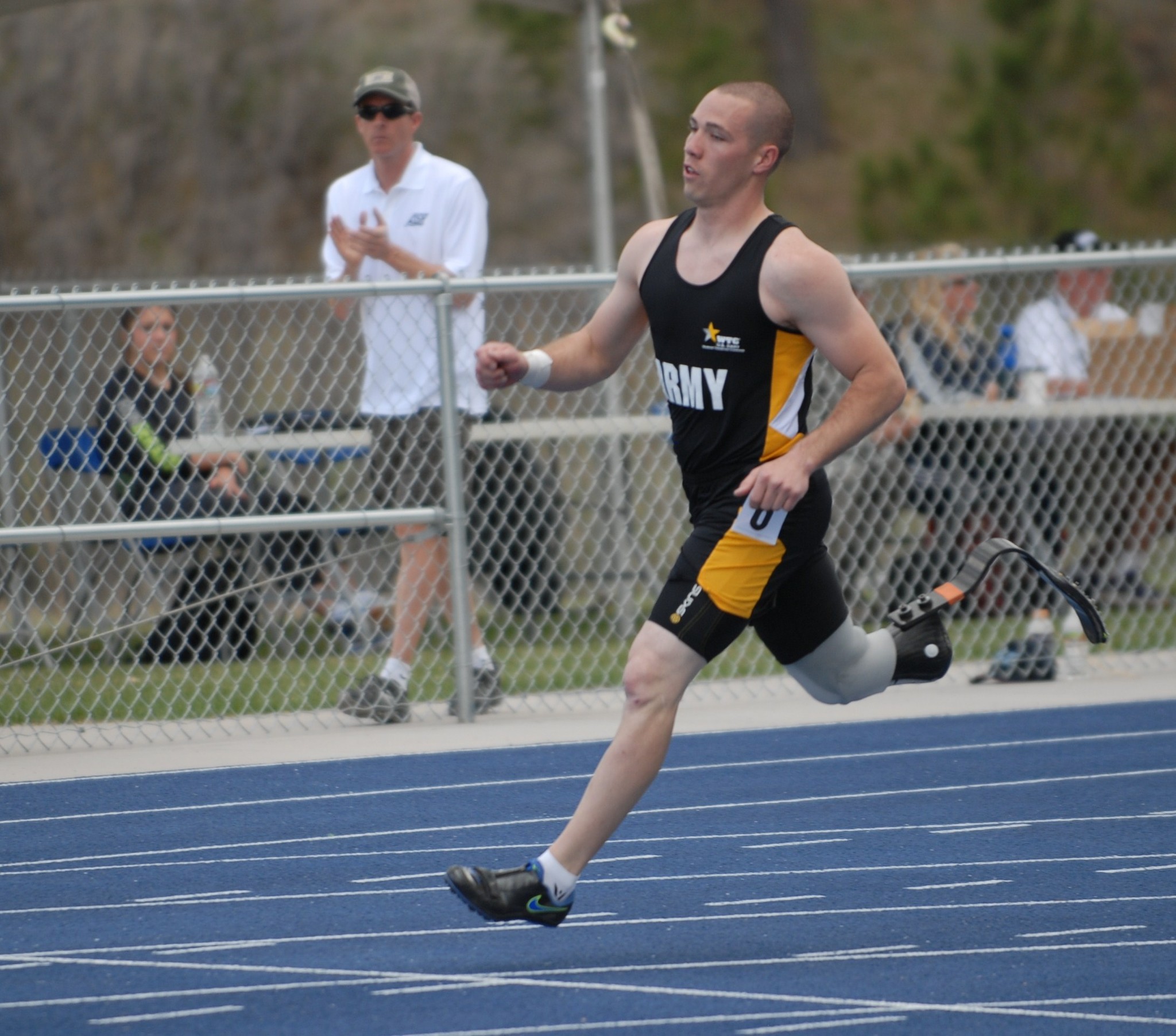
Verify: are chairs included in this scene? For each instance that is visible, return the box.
[38,410,371,659]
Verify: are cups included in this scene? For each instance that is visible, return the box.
[1017,364,1048,406]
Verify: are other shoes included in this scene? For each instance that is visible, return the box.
[447,658,504,717]
[319,591,396,652]
[337,672,411,724]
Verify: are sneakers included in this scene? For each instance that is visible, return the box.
[445,860,572,928]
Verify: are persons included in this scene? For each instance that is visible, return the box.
[822,230,1175,614]
[102,307,396,642]
[320,66,503,724]
[444,81,1109,928]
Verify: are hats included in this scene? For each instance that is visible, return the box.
[1053,228,1118,253]
[351,67,420,110]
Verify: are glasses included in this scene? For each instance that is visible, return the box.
[358,102,415,121]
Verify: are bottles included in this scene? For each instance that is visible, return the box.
[1062,607,1089,676]
[192,353,224,440]
[1029,607,1055,635]
[993,324,1019,380]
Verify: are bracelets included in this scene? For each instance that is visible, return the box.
[519,349,554,387]
